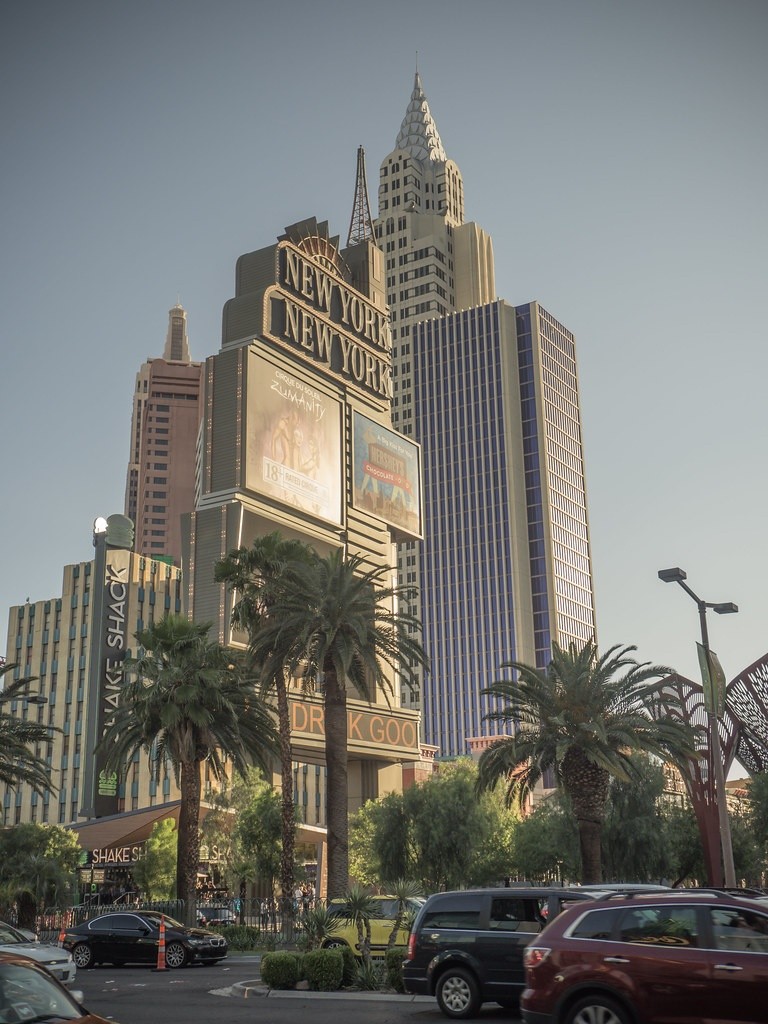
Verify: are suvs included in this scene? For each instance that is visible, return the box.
[520,886,768,1024]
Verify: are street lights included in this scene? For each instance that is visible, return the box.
[660,566,741,884]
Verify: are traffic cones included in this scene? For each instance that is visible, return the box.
[57,925,66,948]
[152,915,172,972]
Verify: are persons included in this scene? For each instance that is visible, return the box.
[296,880,316,920]
[133,897,145,910]
[727,918,765,952]
[261,896,279,930]
[197,881,240,916]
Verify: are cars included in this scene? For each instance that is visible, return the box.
[0,918,122,1024]
[316,892,426,960]
[197,901,241,927]
[62,908,229,968]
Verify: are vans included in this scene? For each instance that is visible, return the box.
[400,884,616,1019]
